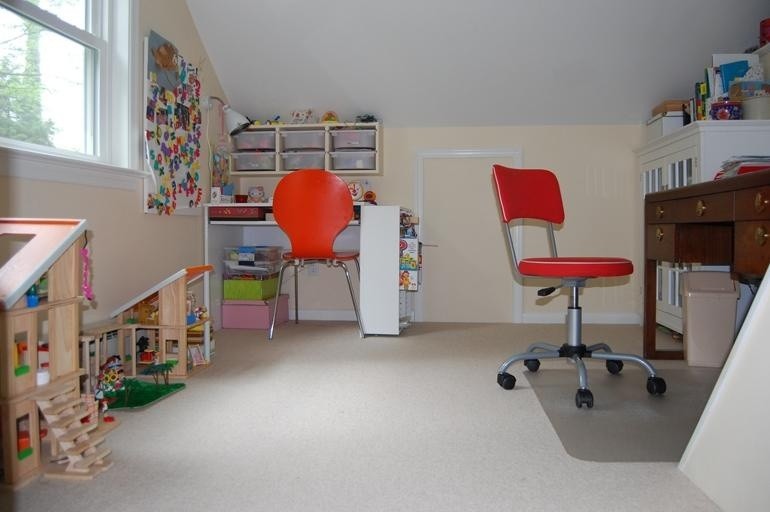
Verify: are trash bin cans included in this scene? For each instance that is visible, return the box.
[679,271,741,368]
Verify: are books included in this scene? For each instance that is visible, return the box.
[681,49,763,124]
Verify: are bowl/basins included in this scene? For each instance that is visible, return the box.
[710,99,743,121]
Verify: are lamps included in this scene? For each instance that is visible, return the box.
[208,96,250,137]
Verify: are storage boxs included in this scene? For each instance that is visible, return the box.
[231,129,377,171]
[218,243,290,329]
[647,100,689,142]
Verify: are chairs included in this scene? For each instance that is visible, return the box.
[489,163,665,408]
[267,171,360,338]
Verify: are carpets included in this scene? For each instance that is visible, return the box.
[523,367,723,463]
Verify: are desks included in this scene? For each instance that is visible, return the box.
[642,170,769,360]
[203,203,400,339]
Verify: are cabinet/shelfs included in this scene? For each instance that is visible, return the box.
[224,122,384,176]
[633,120,770,340]
